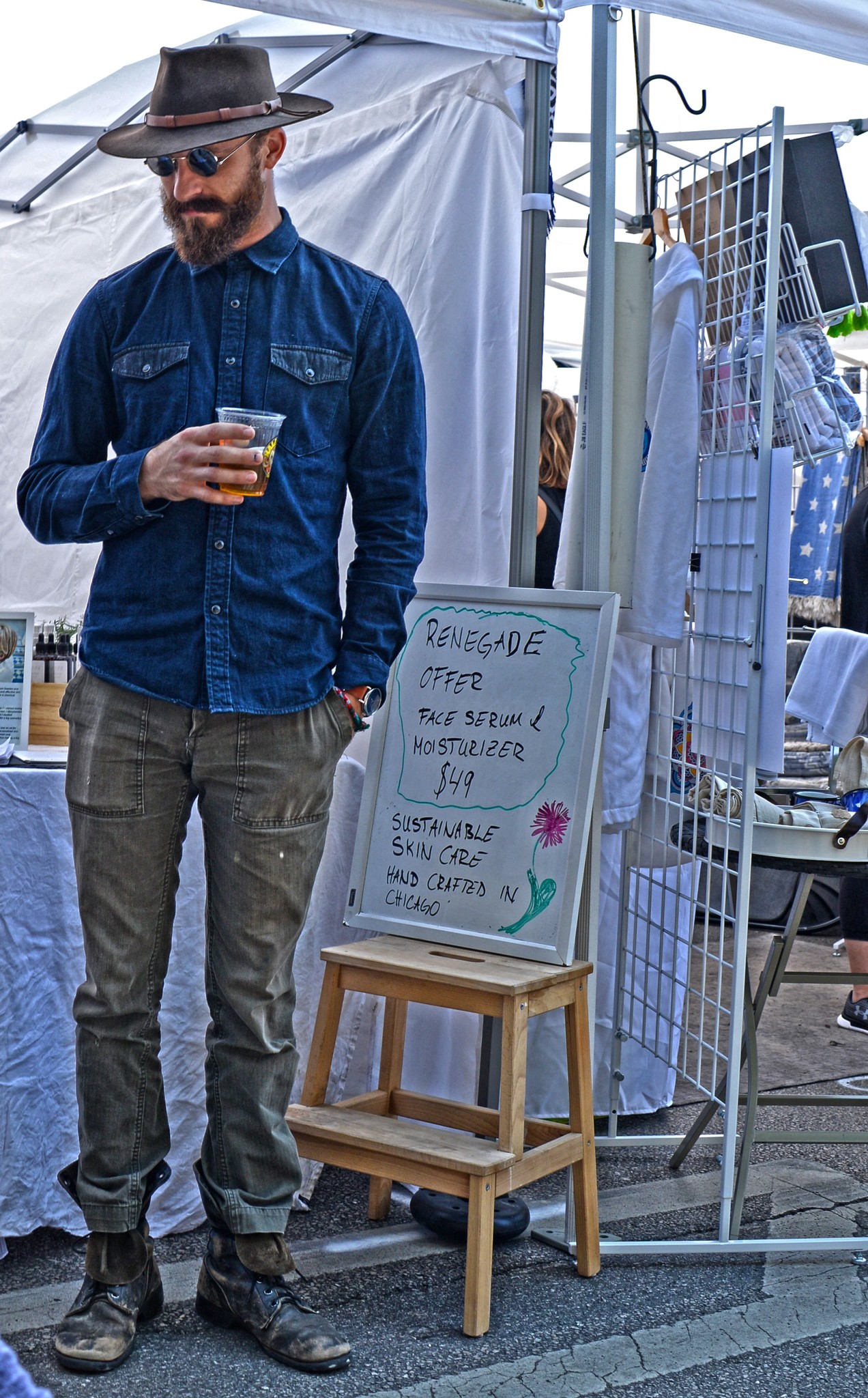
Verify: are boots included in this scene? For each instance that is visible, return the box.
[56,1159,177,1372]
[197,1164,352,1371]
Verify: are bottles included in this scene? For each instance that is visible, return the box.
[35,633,46,655]
[57,633,78,656]
[45,633,56,654]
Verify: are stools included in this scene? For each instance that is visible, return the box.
[284,932,601,1336]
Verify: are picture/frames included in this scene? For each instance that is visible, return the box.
[343,582,620,964]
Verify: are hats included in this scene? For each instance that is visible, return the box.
[96,45,335,159]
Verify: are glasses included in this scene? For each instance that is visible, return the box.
[144,135,261,178]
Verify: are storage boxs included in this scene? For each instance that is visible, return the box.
[675,131,868,345]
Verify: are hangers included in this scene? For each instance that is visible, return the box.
[639,173,697,267]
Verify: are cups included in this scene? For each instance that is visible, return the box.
[215,407,287,496]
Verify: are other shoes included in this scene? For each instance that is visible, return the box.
[837,989,868,1035]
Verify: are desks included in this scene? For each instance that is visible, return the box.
[0,745,366,1257]
[670,819,868,1242]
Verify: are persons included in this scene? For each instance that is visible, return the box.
[533,387,575,591]
[838,490,868,1034]
[14,45,430,1371]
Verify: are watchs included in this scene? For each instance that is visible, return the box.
[346,684,383,718]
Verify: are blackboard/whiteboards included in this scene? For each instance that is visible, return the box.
[339,580,621,967]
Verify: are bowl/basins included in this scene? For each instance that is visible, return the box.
[841,787,868,812]
[793,791,838,805]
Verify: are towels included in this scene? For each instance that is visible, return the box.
[787,626,868,750]
[687,773,868,831]
[831,736,868,794]
